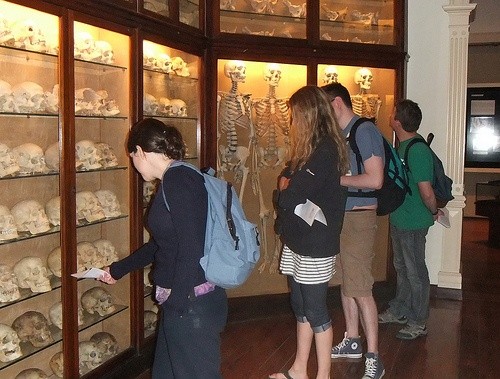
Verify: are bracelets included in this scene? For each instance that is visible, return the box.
[432,209,438,215]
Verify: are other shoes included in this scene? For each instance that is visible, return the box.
[378,305,407,324]
[395,319,428,339]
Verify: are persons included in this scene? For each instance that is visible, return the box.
[267,84,350,379]
[377,100,445,341]
[96,118,229,379]
[320,82,386,379]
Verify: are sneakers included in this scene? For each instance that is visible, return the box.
[331,332,363,358]
[362,352,385,379]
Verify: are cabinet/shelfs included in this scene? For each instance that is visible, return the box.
[0,0,410,379]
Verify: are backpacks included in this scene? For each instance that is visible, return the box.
[404,133,454,209]
[161,159,261,289]
[345,117,412,216]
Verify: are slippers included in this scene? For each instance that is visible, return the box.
[269,369,293,379]
